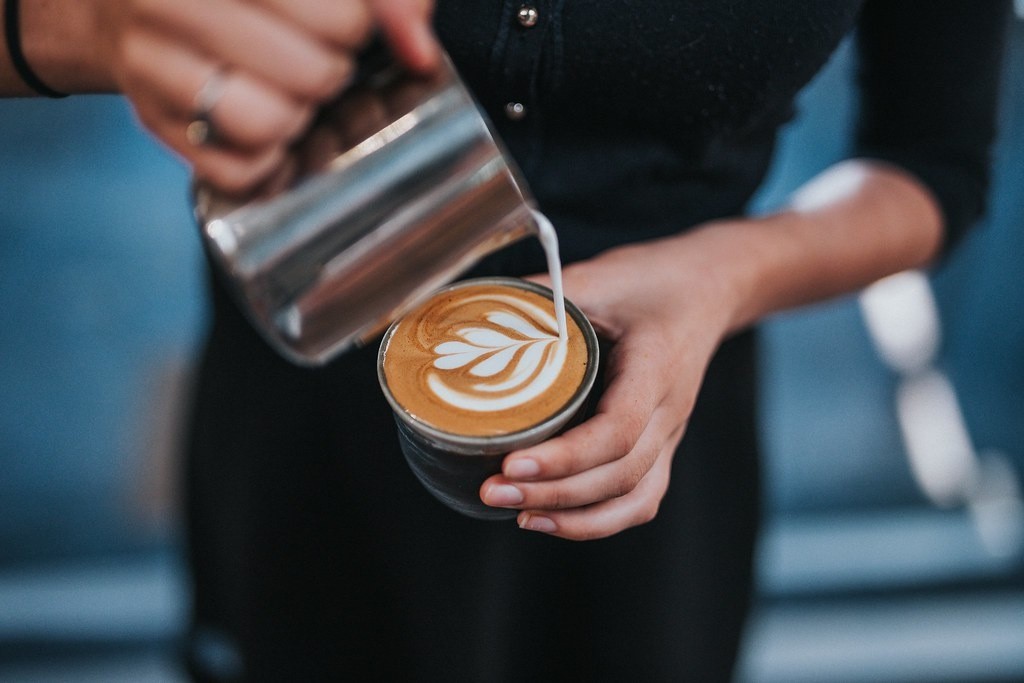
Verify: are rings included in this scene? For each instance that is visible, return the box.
[189,58,233,114]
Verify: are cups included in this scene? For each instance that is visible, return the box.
[378,278,601,522]
[188,44,546,368]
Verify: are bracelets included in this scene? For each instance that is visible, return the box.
[4,0,72,99]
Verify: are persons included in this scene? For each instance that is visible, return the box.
[1,0,1016,682]
